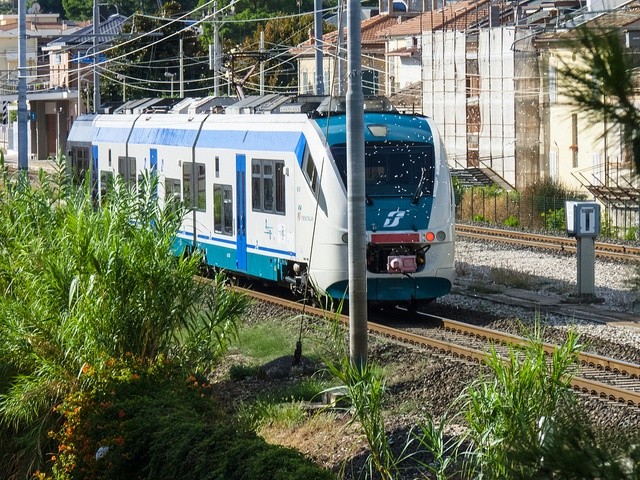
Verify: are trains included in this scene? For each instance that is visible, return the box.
[64,91,456,320]
